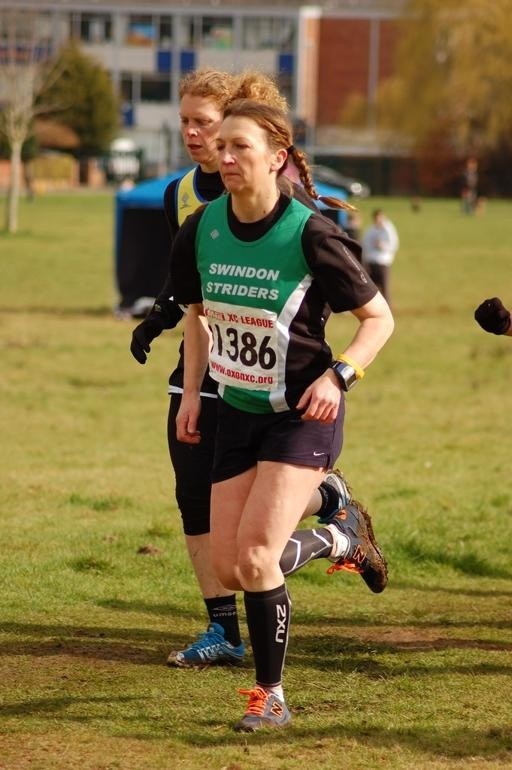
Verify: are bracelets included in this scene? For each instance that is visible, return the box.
[336,354,365,380]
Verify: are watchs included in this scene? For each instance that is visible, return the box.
[330,360,358,392]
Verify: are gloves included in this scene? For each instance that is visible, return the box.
[130,312,166,365]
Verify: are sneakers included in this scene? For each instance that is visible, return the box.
[167,622,245,669]
[235,685,293,733]
[317,469,388,593]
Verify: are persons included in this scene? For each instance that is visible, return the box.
[365,208,400,307]
[347,212,361,240]
[166,98,394,735]
[128,71,354,667]
[461,185,473,214]
